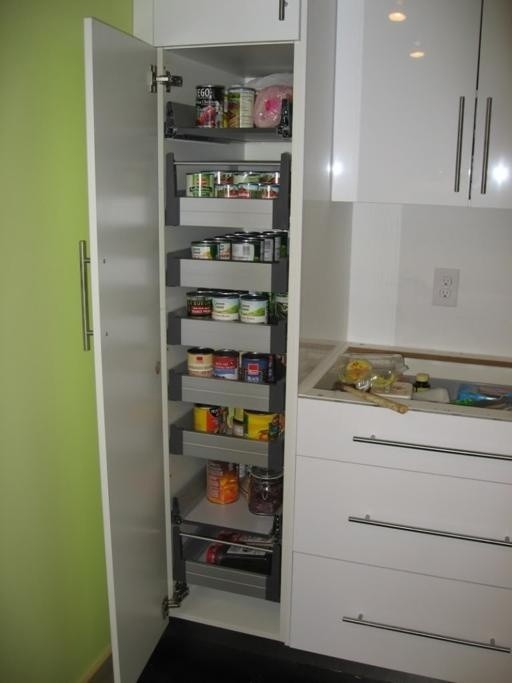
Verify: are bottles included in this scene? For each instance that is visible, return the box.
[246,468,283,517]
[412,372,430,394]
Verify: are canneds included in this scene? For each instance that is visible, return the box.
[193,403,285,440]
[185,287,288,325]
[191,231,287,264]
[186,346,286,383]
[184,169,280,199]
[205,459,285,515]
[195,83,254,130]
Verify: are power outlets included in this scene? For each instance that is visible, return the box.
[430,265,461,308]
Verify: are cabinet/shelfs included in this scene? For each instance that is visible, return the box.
[79,15,336,681]
[330,0,509,210]
[288,395,510,682]
[129,0,336,46]
